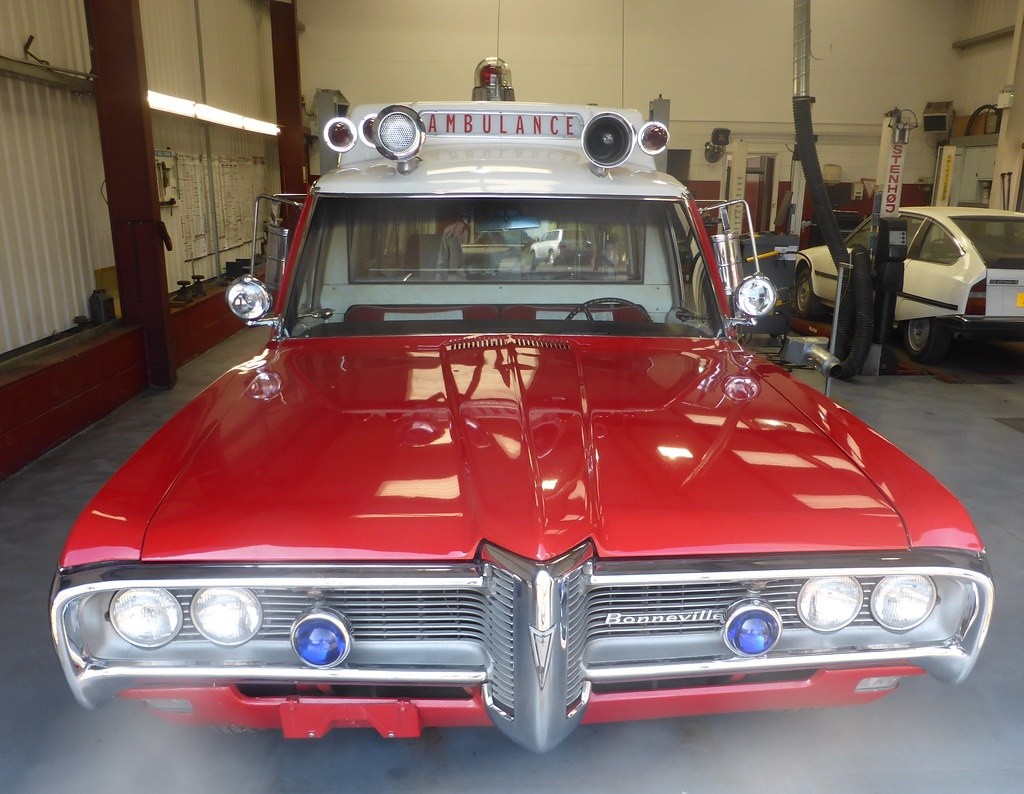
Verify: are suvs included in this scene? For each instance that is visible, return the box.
[527,226,592,267]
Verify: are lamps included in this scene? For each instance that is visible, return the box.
[146,89,280,136]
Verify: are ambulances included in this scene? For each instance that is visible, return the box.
[50,54,999,757]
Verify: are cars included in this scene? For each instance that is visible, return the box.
[793,204,1023,368]
